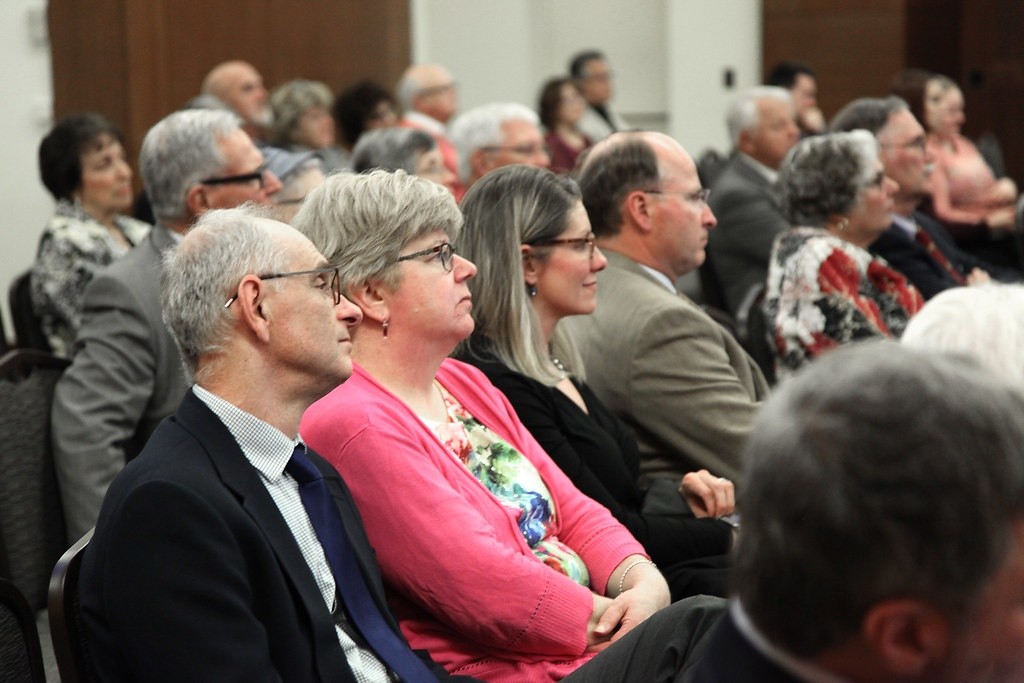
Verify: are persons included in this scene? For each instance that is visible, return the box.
[51,109,278,541]
[693,59,1024,379]
[291,167,670,683]
[85,207,489,683]
[188,51,632,226]
[557,130,773,513]
[30,113,155,359]
[565,283,1024,683]
[449,165,741,605]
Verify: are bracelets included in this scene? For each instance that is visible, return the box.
[619,560,658,593]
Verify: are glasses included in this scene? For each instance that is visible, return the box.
[388,243,462,273]
[530,232,597,260]
[201,160,273,189]
[224,267,341,310]
[642,187,712,204]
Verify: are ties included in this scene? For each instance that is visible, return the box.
[914,224,967,288]
[286,446,438,683]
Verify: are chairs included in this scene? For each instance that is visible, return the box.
[0,269,95,681]
